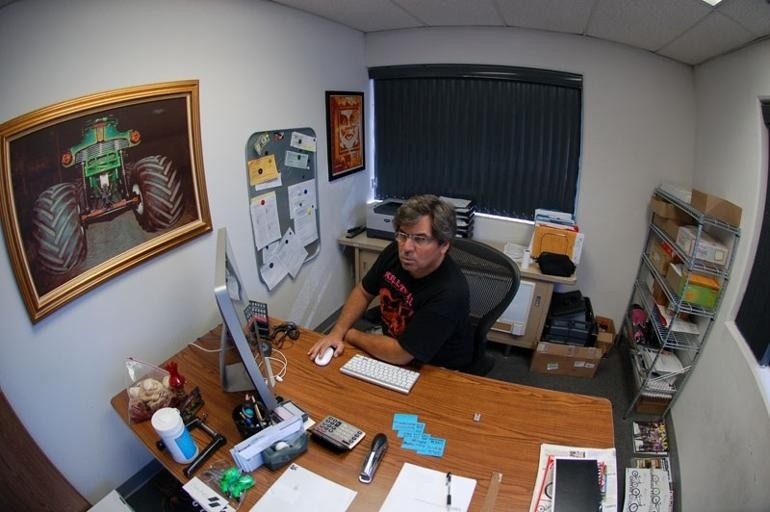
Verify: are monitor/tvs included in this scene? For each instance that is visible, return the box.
[215,225,281,412]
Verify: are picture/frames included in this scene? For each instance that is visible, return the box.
[325,90,365,182]
[0,80,213,325]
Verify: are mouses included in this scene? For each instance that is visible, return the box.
[314,346,334,366]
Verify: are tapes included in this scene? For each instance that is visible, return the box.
[275,441,289,451]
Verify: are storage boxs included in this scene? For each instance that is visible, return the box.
[528,316,616,378]
[647,195,729,308]
[631,378,667,414]
[689,188,743,227]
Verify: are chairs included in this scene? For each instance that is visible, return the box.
[335,237,520,377]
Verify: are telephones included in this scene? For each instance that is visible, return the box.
[226,300,269,337]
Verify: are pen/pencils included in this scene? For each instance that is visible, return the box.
[240,394,265,428]
[445,472,452,512]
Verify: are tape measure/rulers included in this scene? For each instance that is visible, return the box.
[481,472,503,512]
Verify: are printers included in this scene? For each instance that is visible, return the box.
[366,198,408,240]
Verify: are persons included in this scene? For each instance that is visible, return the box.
[306,193,472,374]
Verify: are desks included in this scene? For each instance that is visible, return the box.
[109,312,618,512]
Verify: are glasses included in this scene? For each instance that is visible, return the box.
[393,231,437,247]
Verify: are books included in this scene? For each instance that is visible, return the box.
[438,193,477,241]
[528,416,675,512]
[635,299,698,404]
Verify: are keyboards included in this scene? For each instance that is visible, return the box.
[339,352,420,394]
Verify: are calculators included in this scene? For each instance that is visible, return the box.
[307,415,366,451]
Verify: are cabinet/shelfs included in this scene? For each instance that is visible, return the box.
[337,234,578,349]
[615,186,741,424]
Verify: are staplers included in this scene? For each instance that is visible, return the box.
[358,433,388,483]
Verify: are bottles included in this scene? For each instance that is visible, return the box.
[150,407,200,466]
